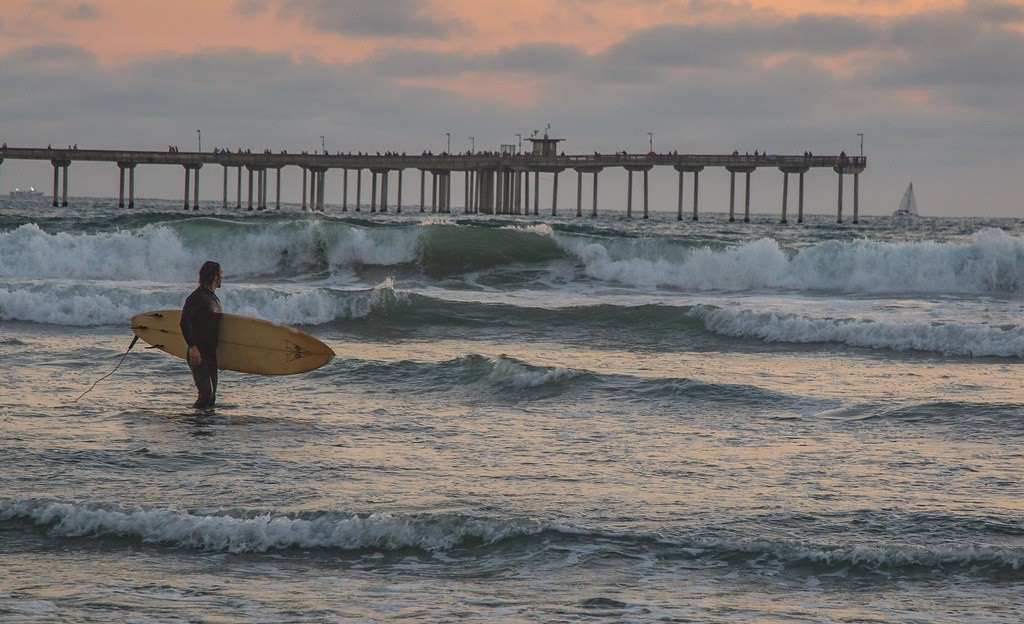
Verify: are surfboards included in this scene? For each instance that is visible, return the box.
[129,304,338,378]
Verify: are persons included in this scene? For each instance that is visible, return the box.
[179,260,224,408]
